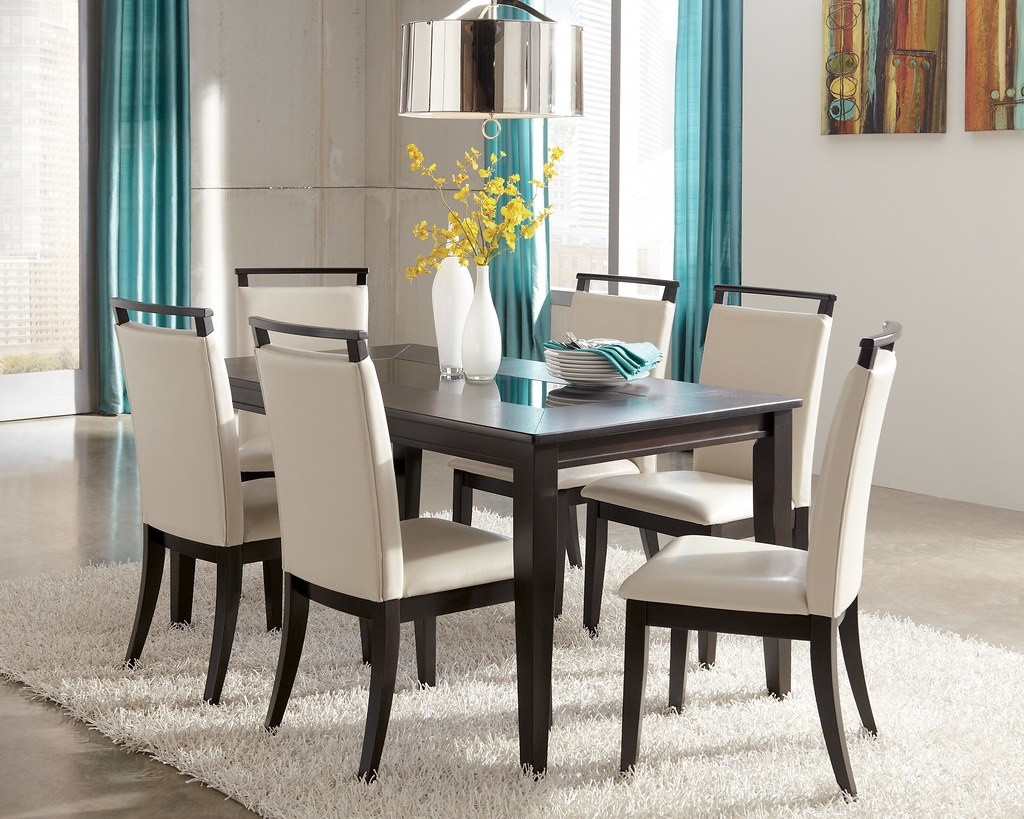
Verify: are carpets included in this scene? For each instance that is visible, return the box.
[0,507,1024,819]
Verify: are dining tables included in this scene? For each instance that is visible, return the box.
[224,343,804,780]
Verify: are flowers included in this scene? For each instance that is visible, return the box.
[406,144,563,279]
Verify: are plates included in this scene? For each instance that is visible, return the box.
[543,335,638,389]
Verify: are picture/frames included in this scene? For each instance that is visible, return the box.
[820,0,1024,136]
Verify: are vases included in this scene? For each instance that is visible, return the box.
[461,265,502,381]
[431,222,474,377]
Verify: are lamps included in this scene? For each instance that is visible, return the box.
[397,0,582,140]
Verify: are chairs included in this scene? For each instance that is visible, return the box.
[618,321,902,803]
[249,316,514,784]
[111,297,281,705]
[448,272,679,619]
[581,285,837,668]
[235,267,370,481]
